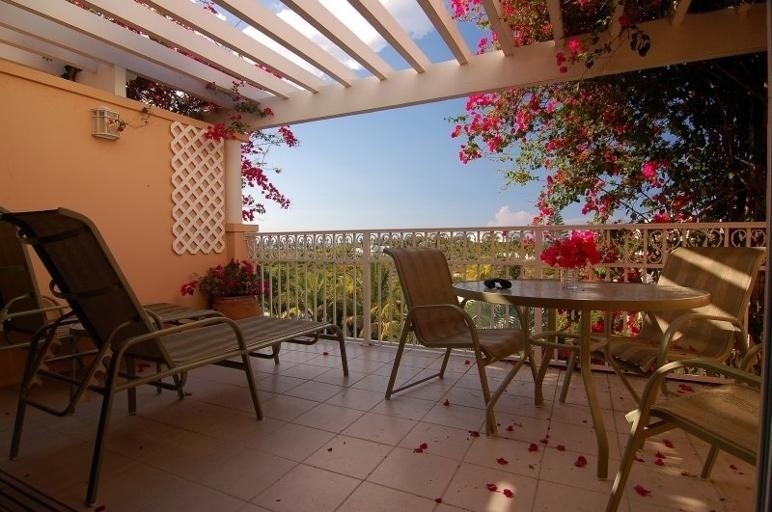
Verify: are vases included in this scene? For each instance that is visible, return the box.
[209,295,263,325]
[559,266,579,291]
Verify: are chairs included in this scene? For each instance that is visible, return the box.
[0,204,229,415]
[604,341,768,512]
[382,246,546,438]
[600,245,767,451]
[1,206,351,509]
[557,244,714,414]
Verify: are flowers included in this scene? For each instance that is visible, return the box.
[537,231,605,270]
[179,254,272,298]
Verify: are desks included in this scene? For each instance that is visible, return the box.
[450,278,712,483]
